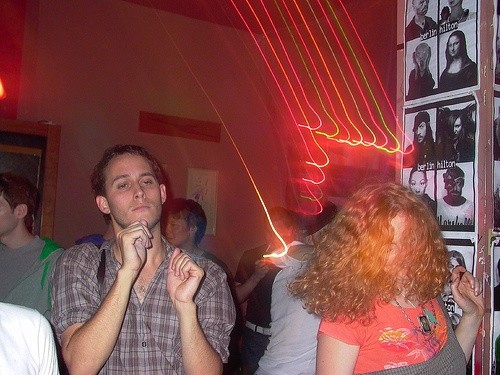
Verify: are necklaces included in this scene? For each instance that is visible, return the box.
[392,289,437,335]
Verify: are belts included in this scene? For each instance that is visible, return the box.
[245,321,272,335]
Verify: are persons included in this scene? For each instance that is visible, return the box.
[285,182,485,375]
[403,0,478,226]
[0,145,332,374]
